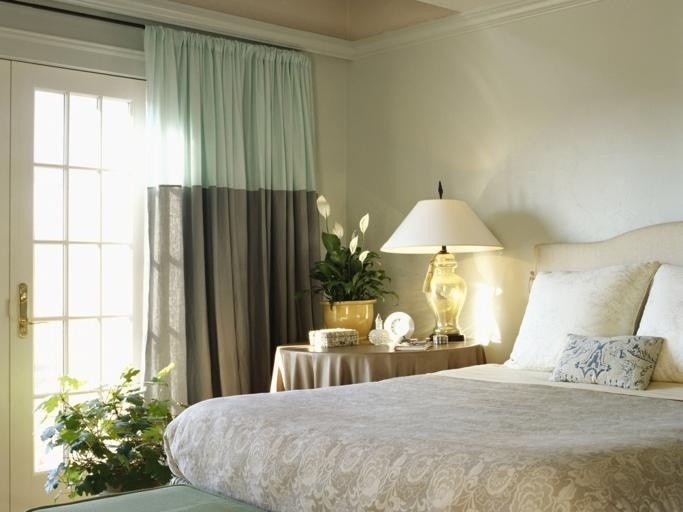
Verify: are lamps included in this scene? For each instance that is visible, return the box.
[380,181,504,342]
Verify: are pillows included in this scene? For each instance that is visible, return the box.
[502,261,683,392]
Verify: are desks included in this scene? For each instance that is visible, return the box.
[272,340,484,391]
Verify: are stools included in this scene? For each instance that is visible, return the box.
[24,483,276,512]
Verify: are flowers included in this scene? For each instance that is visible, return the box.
[293,193,401,310]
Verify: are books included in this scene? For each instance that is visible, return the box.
[394,341,432,351]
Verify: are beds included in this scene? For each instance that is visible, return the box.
[159,221,682,512]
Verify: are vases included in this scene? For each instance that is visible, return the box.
[320,299,378,341]
